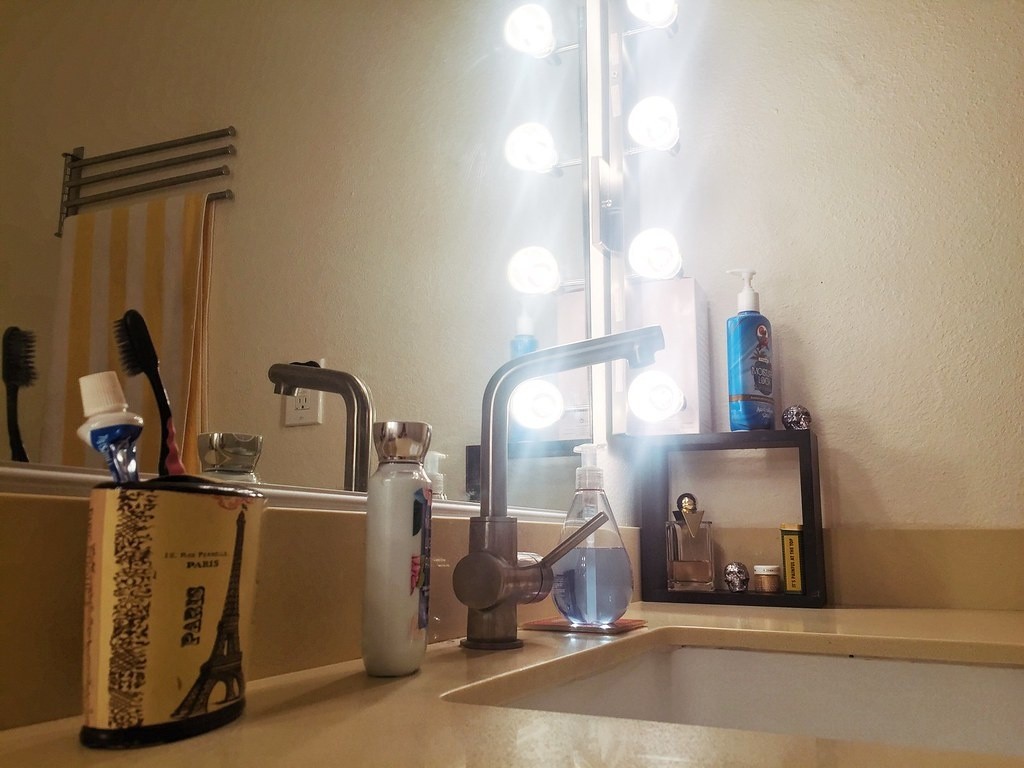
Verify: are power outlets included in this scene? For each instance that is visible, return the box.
[282,354,329,428]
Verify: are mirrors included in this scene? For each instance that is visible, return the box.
[0,0,611,526]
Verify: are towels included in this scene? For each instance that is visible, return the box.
[36,188,219,475]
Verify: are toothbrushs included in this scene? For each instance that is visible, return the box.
[111,307,190,478]
[1,325,41,462]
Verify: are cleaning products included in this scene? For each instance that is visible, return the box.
[725,267,775,432]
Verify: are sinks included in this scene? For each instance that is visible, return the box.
[439,625,1023,760]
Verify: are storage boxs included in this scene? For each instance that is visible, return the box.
[466,437,591,503]
[548,292,591,438]
[621,274,712,435]
[639,429,829,608]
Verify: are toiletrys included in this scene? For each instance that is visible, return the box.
[549,440,636,627]
[421,449,448,506]
[75,370,145,482]
[360,418,433,679]
[195,430,264,484]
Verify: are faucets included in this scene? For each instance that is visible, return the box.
[266,361,374,495]
[453,324,667,643]
